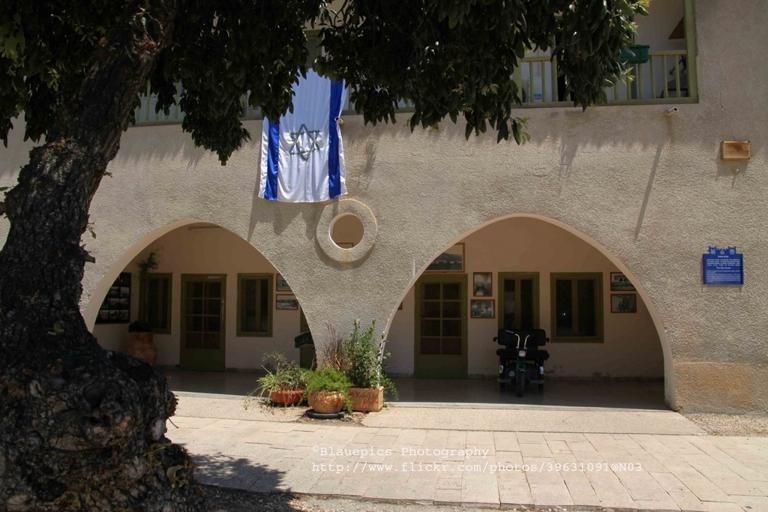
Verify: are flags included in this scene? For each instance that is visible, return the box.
[258,66,348,203]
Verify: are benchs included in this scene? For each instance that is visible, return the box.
[498,328,549,361]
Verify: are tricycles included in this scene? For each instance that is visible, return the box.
[492,327,549,398]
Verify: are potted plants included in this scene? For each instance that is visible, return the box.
[241,317,401,417]
[126,319,158,364]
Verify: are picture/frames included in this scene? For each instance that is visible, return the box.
[423,242,465,272]
[469,271,496,318]
[95,272,131,324]
[275,272,299,311]
[609,272,637,313]
